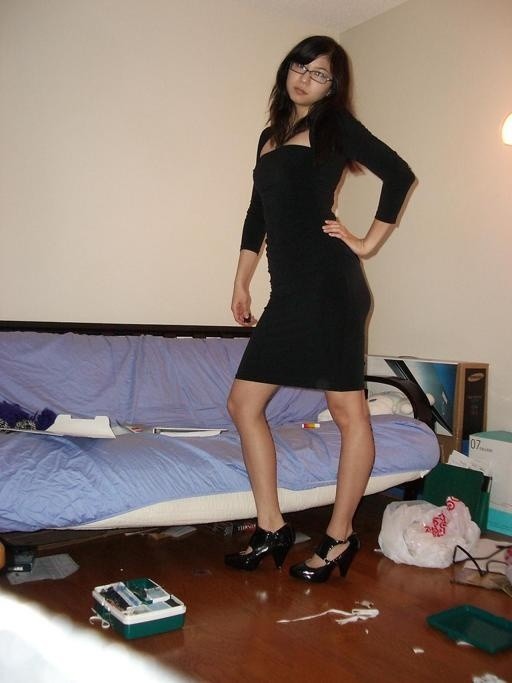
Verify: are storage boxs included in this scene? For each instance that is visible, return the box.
[365,354,512,540]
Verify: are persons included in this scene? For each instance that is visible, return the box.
[223,36,416,582]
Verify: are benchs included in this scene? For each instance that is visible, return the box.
[0,319,433,568]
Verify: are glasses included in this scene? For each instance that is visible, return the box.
[289,62,334,85]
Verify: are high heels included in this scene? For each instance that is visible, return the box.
[225,523,295,571]
[290,533,360,582]
